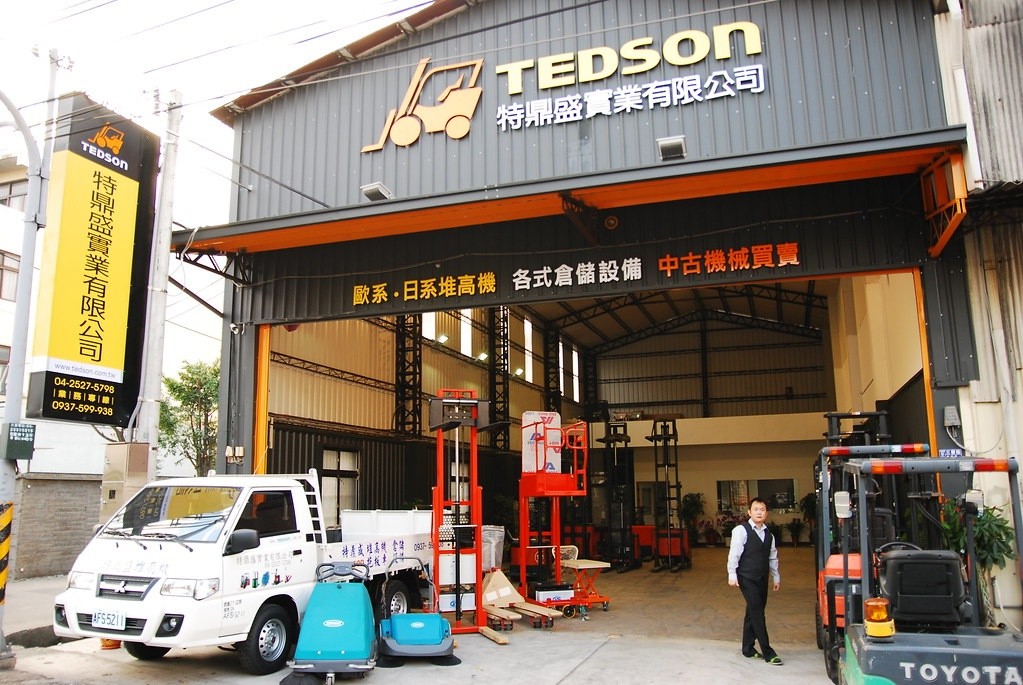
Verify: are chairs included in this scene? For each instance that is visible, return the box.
[881,550,966,634]
[246,500,289,534]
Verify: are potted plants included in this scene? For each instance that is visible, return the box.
[784,521,809,547]
[680,492,706,547]
[703,528,721,545]
[767,521,783,547]
[721,521,737,547]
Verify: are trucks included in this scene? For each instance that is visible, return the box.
[48,466,453,676]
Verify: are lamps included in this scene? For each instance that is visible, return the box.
[223,446,245,466]
[358,180,394,201]
[475,352,488,363]
[512,368,523,379]
[655,135,689,160]
[432,334,450,349]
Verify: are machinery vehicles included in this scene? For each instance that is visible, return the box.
[812,441,1023,684]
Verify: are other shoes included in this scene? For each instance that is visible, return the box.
[766,656,783,665]
[746,653,765,659]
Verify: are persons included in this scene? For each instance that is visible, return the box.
[727,498,784,664]
